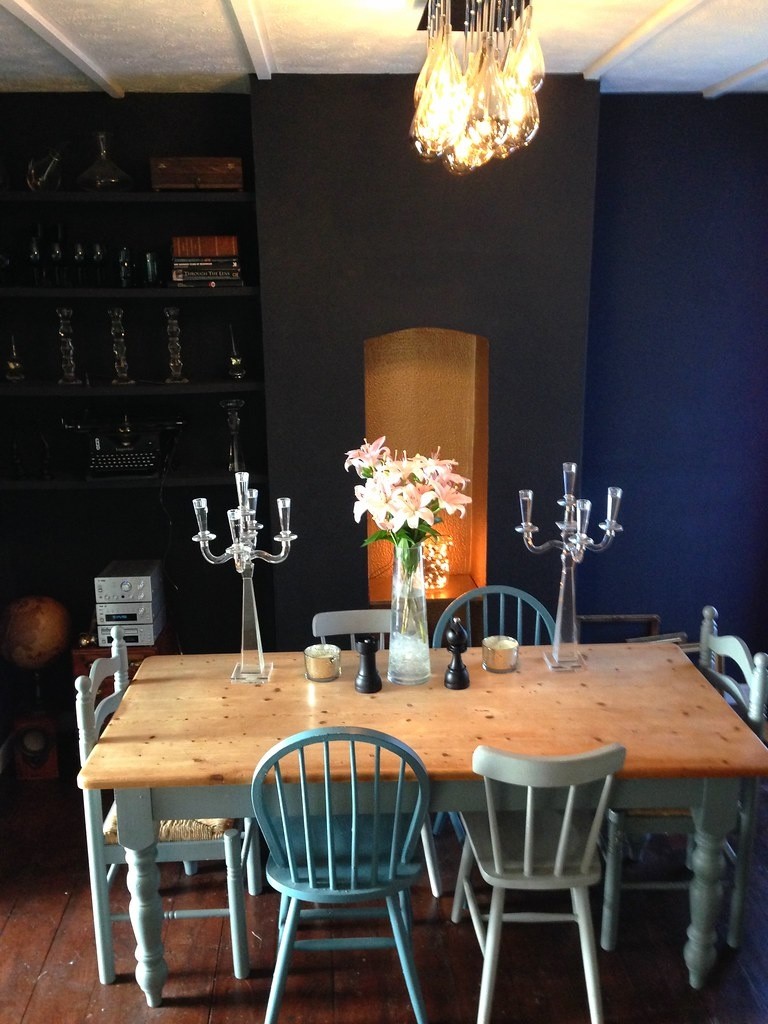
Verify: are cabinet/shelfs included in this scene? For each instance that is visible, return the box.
[0,88,268,500]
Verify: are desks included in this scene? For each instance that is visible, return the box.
[76,641,768,990]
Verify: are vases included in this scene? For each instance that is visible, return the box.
[387,546,433,686]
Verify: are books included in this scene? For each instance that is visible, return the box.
[172,235,243,286]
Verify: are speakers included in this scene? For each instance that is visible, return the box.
[13,716,59,780]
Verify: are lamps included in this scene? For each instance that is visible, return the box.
[410,0,546,176]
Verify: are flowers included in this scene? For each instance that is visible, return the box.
[344,437,471,548]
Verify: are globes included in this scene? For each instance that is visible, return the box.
[3,595,73,716]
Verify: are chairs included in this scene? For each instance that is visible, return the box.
[74,626,261,986]
[594,607,768,948]
[250,727,431,1024]
[451,746,626,1024]
[431,583,556,909]
[312,609,444,899]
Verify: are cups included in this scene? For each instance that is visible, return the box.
[142,242,160,283]
[482,634,519,674]
[304,644,341,683]
[116,247,133,280]
